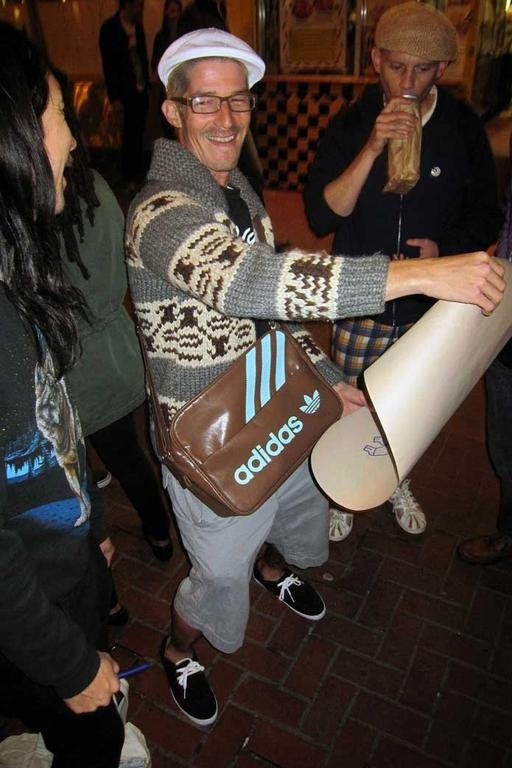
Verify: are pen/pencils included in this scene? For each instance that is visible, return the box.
[118,662,156,679]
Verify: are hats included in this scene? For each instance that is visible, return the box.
[157,25,268,93]
[374,0,462,63]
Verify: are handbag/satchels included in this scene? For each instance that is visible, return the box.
[1,676,152,768]
[159,323,346,522]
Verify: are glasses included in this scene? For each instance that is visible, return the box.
[168,90,258,116]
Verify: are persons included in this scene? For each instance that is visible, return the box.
[457,202,511,566]
[303,3,492,541]
[0,0,225,768]
[127,29,506,726]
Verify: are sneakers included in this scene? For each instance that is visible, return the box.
[251,556,327,621]
[328,506,355,543]
[388,479,427,536]
[158,633,219,727]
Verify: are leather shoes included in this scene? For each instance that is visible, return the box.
[147,534,174,563]
[456,528,512,567]
[107,600,129,627]
